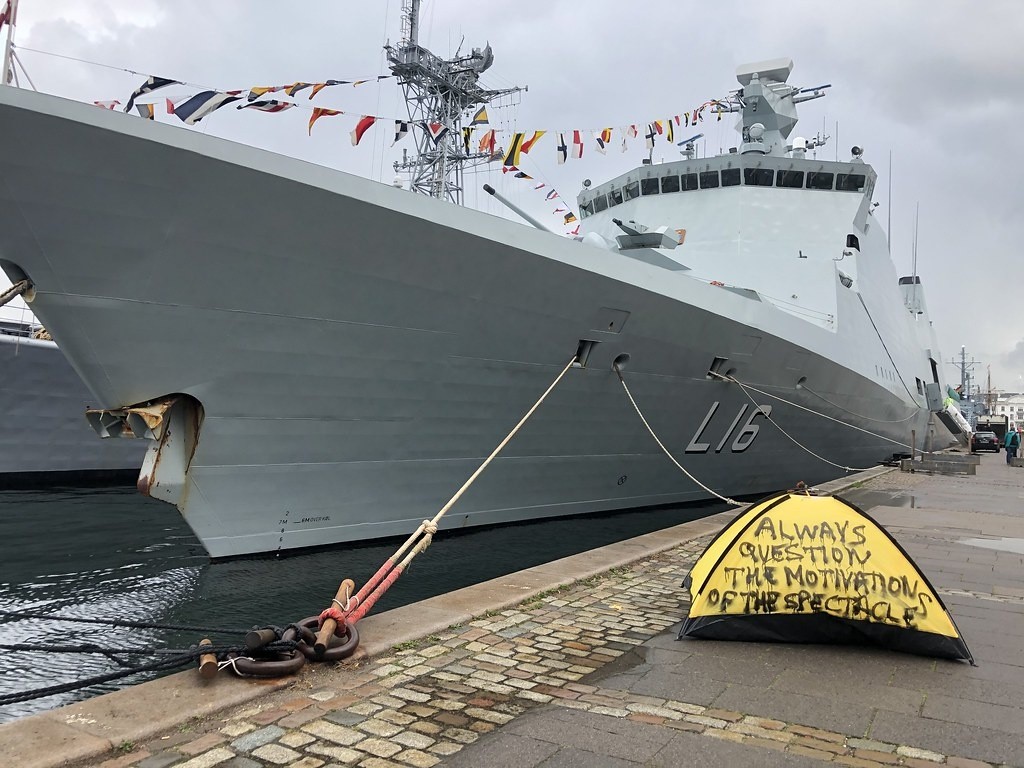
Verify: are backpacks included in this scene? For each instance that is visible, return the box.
[1010,433,1018,445]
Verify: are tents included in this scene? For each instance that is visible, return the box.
[674,481,977,667]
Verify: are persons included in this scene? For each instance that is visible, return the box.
[1003,427,1020,465]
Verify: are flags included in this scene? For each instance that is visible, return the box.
[521,131,546,154]
[504,132,526,166]
[236,99,299,113]
[173,90,247,127]
[666,119,673,142]
[350,115,378,146]
[692,110,699,126]
[571,131,585,158]
[645,122,656,148]
[556,132,568,165]
[592,132,606,155]
[563,212,577,226]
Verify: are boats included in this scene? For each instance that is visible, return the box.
[0,0,1005,558]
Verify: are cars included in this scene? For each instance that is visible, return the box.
[972,432,1000,452]
